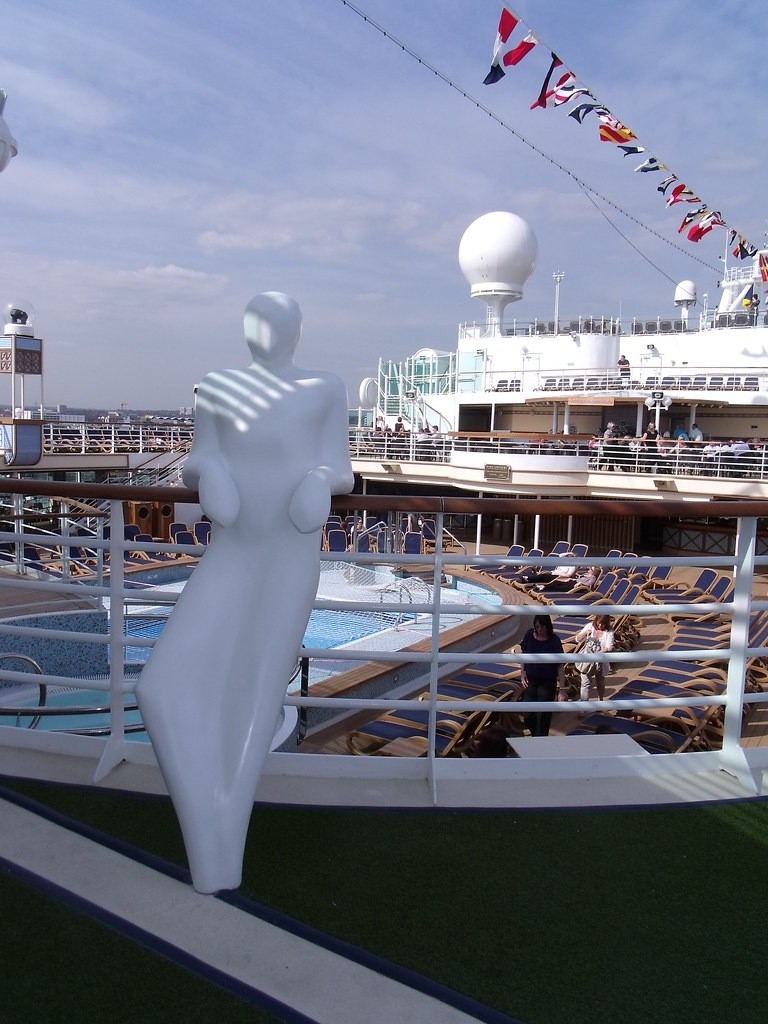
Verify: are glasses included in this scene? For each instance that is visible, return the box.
[590,567,596,573]
[534,623,547,630]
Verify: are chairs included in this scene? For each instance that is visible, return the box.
[0,522,213,581]
[537,323,559,334]
[631,319,687,333]
[322,515,453,554]
[718,315,750,327]
[350,435,445,462]
[567,318,622,334]
[43,426,194,451]
[347,542,768,757]
[605,444,768,477]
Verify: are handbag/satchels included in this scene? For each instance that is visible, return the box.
[574,621,597,677]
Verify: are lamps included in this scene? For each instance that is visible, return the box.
[10,309,27,324]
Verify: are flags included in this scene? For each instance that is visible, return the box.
[484,8,768,282]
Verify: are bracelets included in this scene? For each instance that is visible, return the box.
[558,688,565,691]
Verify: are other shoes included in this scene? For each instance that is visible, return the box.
[516,575,527,584]
[532,583,540,593]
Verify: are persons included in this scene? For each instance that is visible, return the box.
[475,552,614,757]
[618,355,630,379]
[748,290,768,326]
[372,416,763,478]
